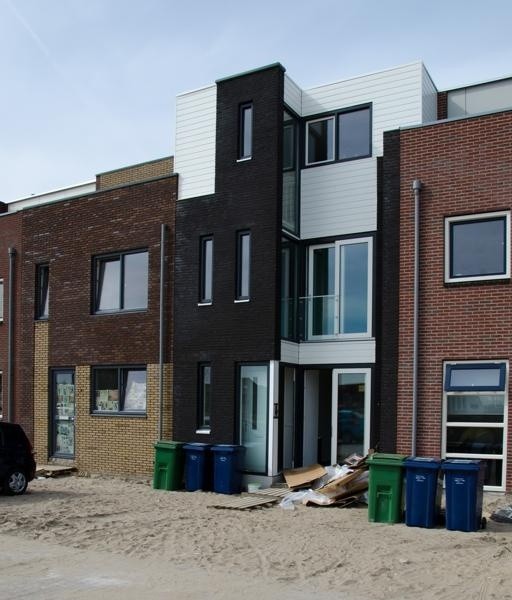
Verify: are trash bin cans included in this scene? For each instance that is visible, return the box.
[364,452,487,532]
[153,441,247,495]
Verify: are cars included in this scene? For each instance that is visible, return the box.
[0,419,36,495]
